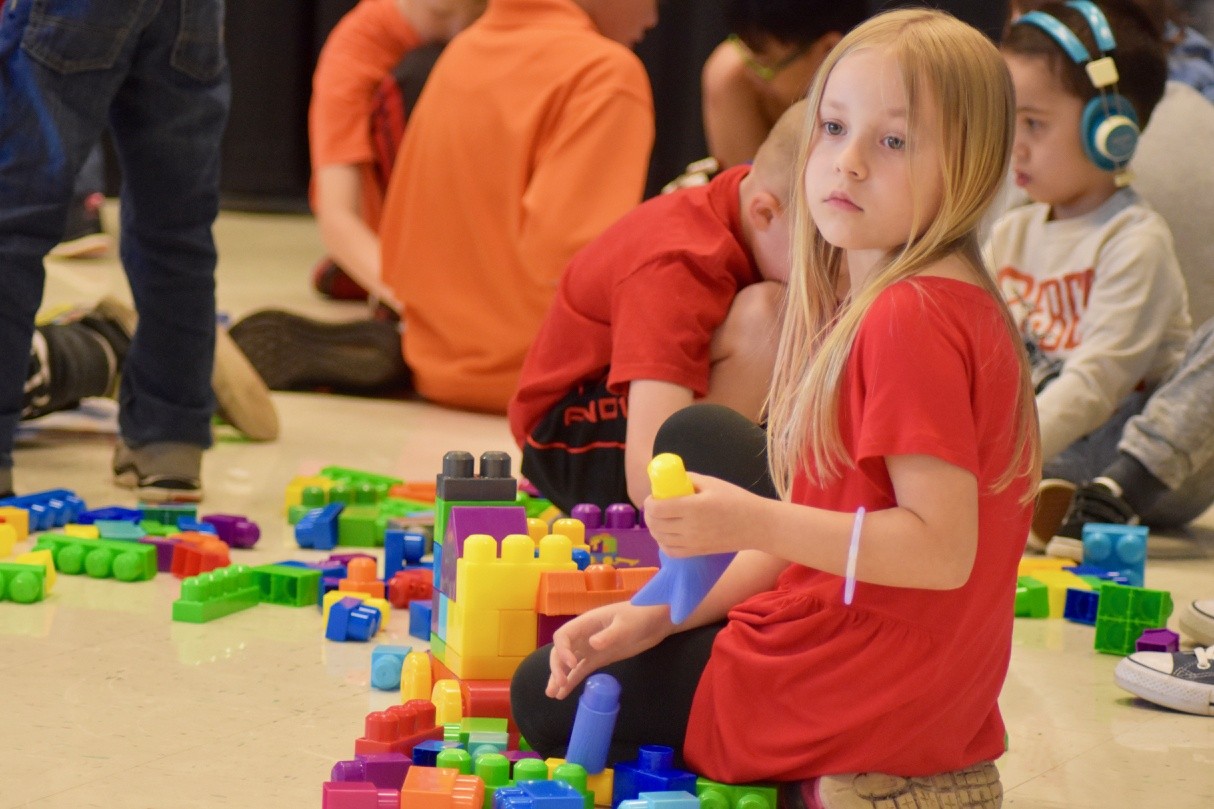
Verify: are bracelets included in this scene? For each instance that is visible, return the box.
[823,502,868,608]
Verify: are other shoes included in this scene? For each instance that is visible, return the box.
[81,295,139,400]
[112,440,205,502]
[800,760,1003,809]
[227,309,412,397]
[208,322,282,444]
[48,198,112,259]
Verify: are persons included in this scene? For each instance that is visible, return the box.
[226,0,664,421]
[505,88,849,521]
[1113,592,1214,722]
[980,2,1214,564]
[695,6,873,173]
[0,0,233,512]
[45,124,116,264]
[8,285,283,450]
[511,4,1049,806]
[283,0,500,323]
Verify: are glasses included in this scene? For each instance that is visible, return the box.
[726,28,826,82]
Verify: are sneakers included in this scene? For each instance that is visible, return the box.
[1179,599,1214,646]
[1114,645,1214,718]
[1025,478,1077,553]
[1045,475,1140,564]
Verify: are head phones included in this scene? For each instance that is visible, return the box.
[1015,0,1140,186]
